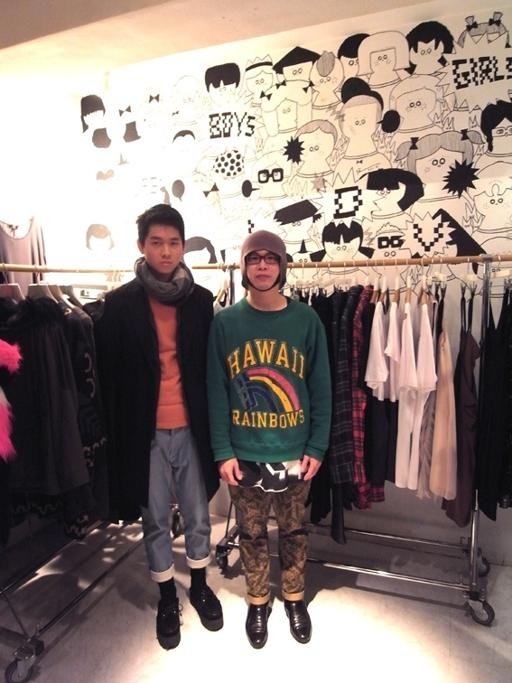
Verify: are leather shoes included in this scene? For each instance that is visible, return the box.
[188,585,225,632]
[283,600,313,644]
[246,597,270,649]
[155,596,180,651]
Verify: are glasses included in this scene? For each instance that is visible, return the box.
[246,252,279,265]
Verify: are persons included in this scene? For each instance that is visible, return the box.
[93,202,225,651]
[204,229,335,650]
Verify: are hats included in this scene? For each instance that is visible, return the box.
[241,229,287,289]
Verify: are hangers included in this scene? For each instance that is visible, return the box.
[210,254,512,305]
[0,262,127,309]
